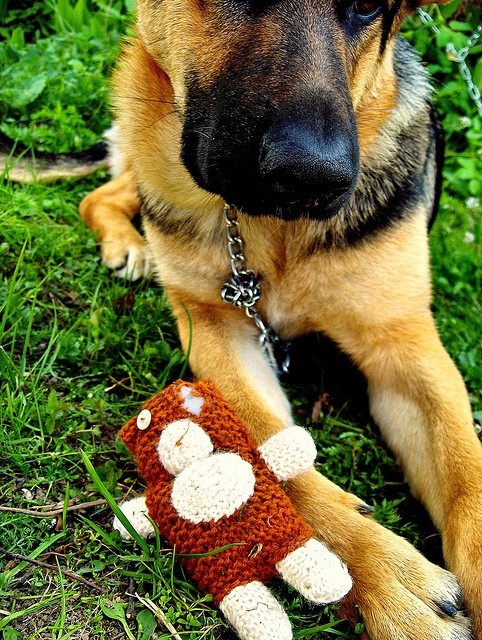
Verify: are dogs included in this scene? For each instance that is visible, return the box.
[77,1,482,640]
